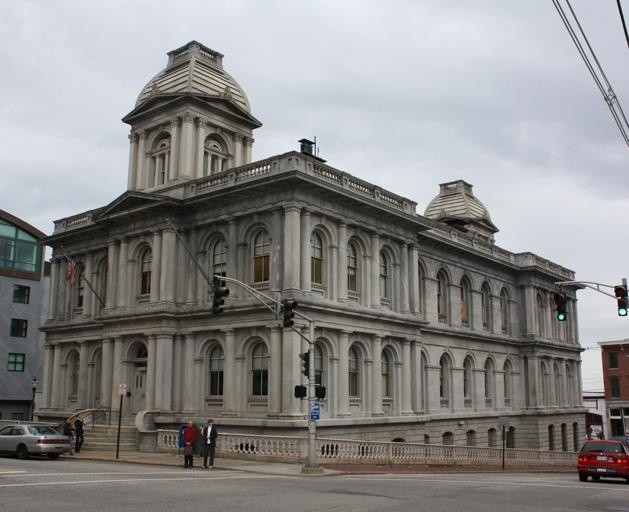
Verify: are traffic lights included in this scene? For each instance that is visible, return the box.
[315,387,325,398]
[299,350,310,377]
[294,385,306,398]
[210,284,230,316]
[284,297,298,328]
[554,291,567,321]
[614,284,628,316]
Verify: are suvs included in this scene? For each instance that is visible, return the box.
[577,438,629,484]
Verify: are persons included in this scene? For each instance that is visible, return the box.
[75,414,84,453]
[183,420,198,468]
[202,419,217,469]
[62,417,75,456]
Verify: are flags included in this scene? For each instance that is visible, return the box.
[66,258,77,286]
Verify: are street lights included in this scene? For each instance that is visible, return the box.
[30,377,39,421]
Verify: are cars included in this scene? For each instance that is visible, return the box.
[0,424,73,459]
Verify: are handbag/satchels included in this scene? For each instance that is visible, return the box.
[184,446,193,456]
